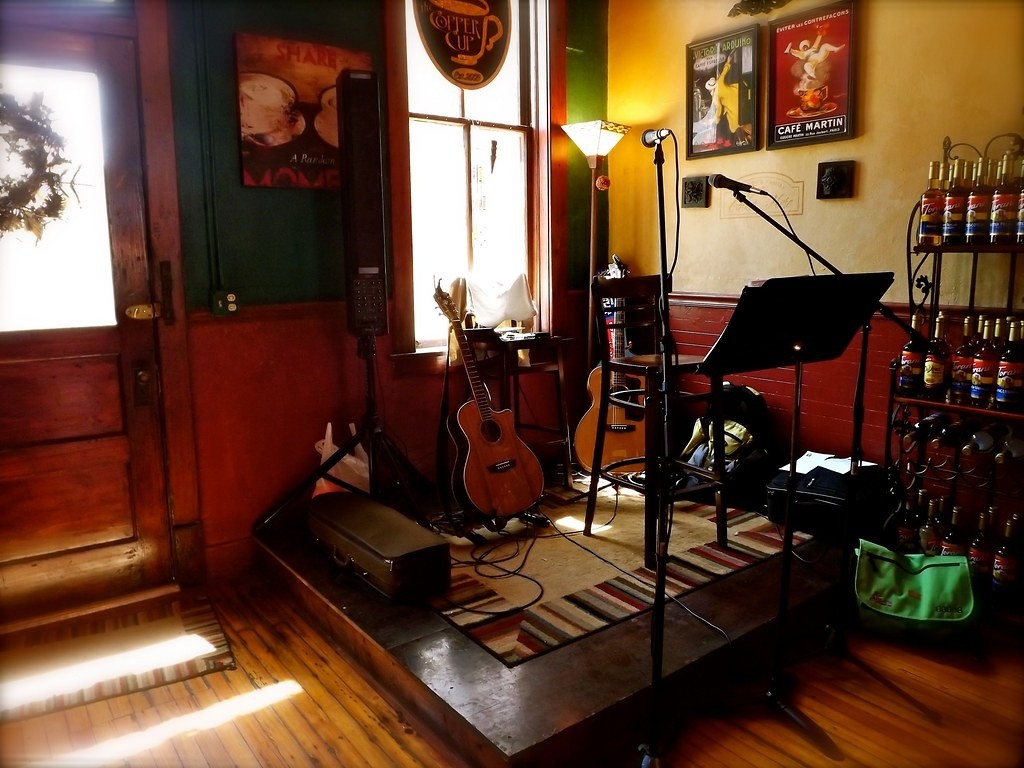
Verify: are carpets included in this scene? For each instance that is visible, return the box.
[428,466,814,667]
[0,587,237,725]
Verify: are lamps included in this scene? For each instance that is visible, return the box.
[561,120,632,414]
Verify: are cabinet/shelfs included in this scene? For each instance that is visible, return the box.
[883,135,1024,627]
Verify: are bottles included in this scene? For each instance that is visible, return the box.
[934,496,948,536]
[918,499,939,554]
[966,512,992,580]
[896,492,918,553]
[939,507,965,555]
[991,520,1019,593]
[919,155,1024,246]
[1012,513,1024,592]
[931,418,980,449]
[904,413,951,443]
[899,315,923,397]
[916,489,928,524]
[995,429,1024,464]
[951,315,1024,412]
[962,423,1010,456]
[924,311,950,401]
[988,507,1001,545]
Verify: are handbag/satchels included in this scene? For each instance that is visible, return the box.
[852,536,976,631]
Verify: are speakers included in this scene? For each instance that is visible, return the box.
[336,67,396,339]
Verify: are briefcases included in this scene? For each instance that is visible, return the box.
[766,453,878,543]
[305,488,451,603]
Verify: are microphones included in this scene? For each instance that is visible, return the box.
[709,174,769,195]
[642,128,670,148]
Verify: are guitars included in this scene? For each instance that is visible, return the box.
[433,283,546,517]
[574,254,649,478]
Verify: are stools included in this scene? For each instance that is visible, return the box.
[583,274,727,569]
[465,274,574,491]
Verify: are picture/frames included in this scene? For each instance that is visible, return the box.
[686,24,760,160]
[765,0,854,151]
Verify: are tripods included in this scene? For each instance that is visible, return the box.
[697,195,942,761]
[256,330,435,536]
